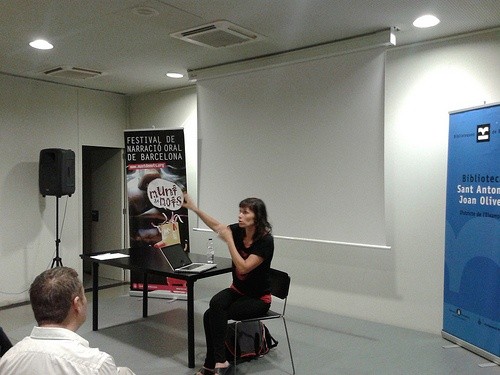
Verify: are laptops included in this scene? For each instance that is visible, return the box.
[160,243,217,273]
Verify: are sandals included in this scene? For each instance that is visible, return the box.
[194,361,237,375]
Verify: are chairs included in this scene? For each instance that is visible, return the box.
[225,269,295,375]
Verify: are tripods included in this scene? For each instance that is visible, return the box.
[51,196,63,269]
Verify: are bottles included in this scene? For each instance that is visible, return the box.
[207,238,215,264]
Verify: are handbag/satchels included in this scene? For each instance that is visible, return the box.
[225,320,278,365]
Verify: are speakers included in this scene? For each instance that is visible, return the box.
[39,149,75,198]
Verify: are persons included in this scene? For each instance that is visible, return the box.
[181,190,274,375]
[0,267,134,375]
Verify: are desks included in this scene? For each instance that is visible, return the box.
[79,248,233,369]
